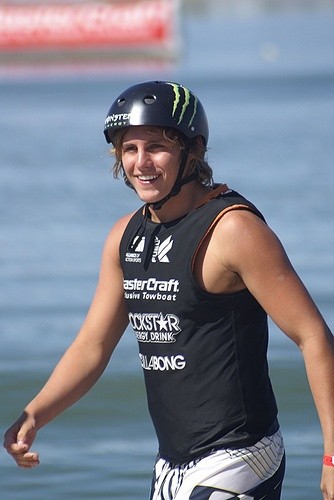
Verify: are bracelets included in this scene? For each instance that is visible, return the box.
[322,454,334,467]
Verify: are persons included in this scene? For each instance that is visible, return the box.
[2,79,334,500]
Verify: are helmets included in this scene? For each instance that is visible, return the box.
[102,81,209,147]
